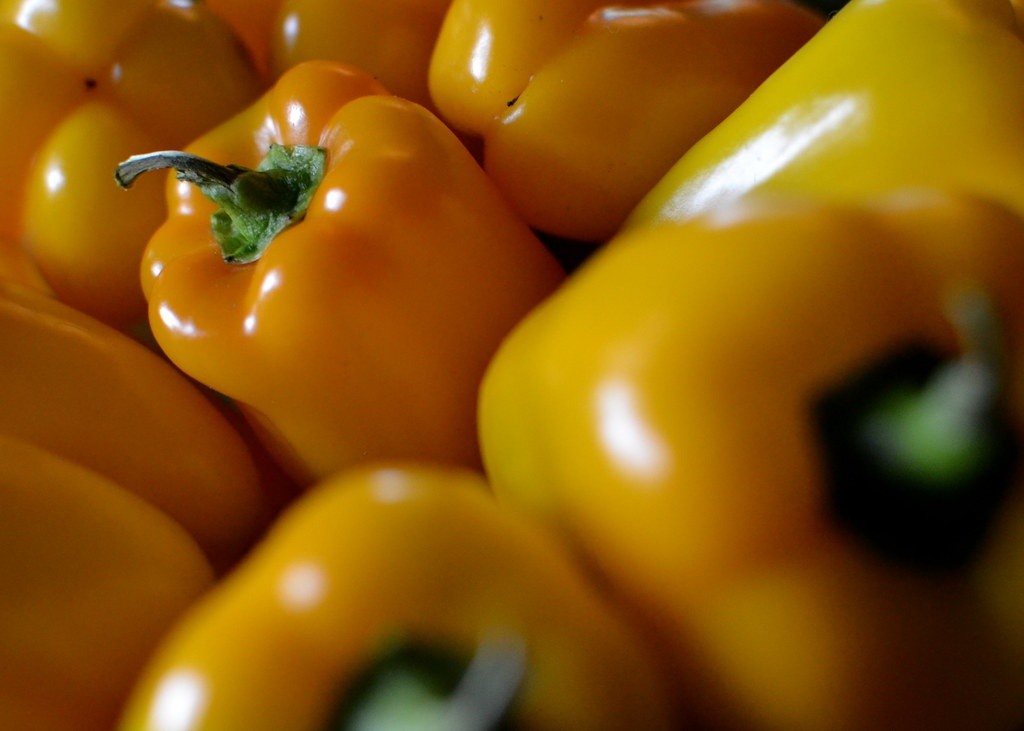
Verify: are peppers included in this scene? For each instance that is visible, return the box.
[0,0,1024,731]
